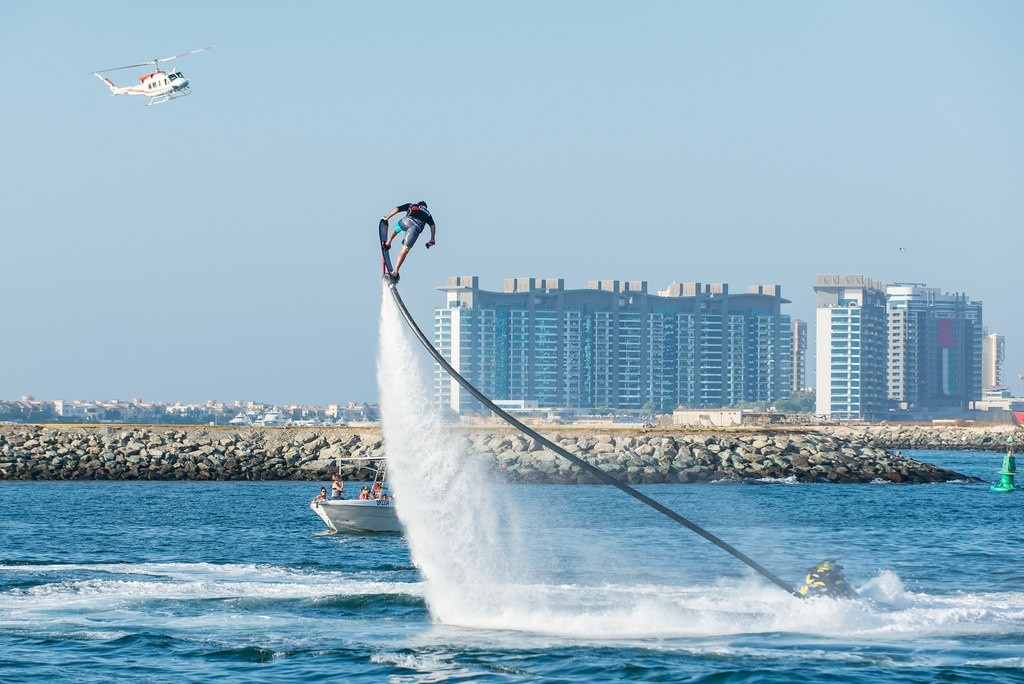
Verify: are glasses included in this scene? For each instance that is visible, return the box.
[322,491,326,493]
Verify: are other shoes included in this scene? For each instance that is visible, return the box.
[389,270,399,277]
[382,241,391,251]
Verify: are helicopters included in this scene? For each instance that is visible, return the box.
[89,46,215,106]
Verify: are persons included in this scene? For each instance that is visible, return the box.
[313,487,326,501]
[359,486,394,500]
[331,474,347,500]
[381,200,436,278]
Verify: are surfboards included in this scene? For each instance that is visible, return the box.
[379,216,401,285]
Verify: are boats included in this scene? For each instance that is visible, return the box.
[307,456,402,534]
